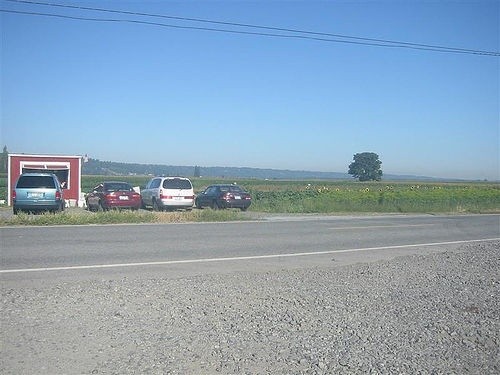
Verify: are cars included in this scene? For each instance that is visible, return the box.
[195,184,252,211]
[85,181,142,211]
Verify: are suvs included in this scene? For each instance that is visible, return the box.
[13,170,65,214]
[141,176,195,211]
